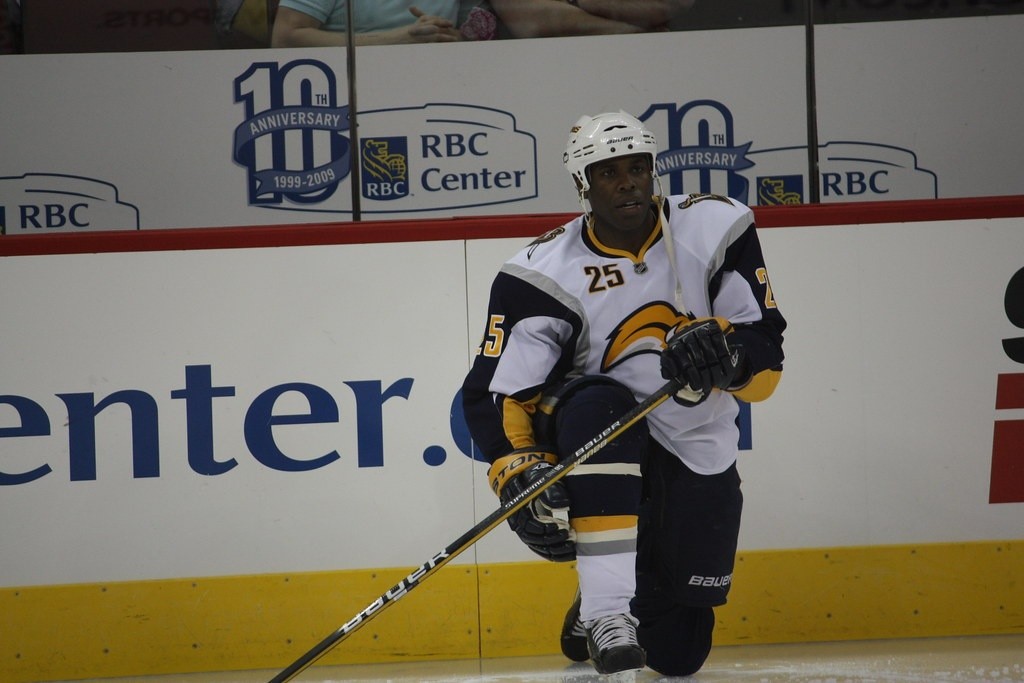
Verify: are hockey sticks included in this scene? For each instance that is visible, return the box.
[267,379,679,683]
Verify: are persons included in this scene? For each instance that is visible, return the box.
[269,0,696,47]
[461,110,789,683]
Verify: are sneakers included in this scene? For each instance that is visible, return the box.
[583,611,647,683]
[560,582,590,662]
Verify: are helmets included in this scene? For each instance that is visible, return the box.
[563,109,658,199]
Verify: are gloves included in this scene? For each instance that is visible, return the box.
[487,446,578,563]
[659,316,745,392]
